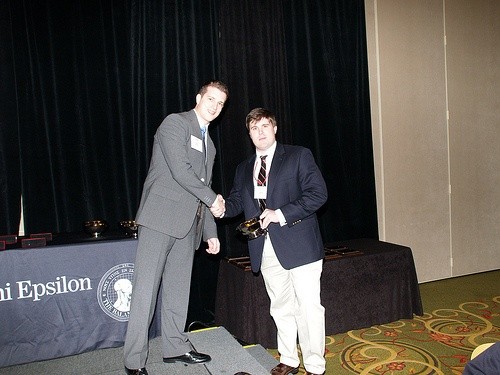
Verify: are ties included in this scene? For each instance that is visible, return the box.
[257,155,268,214]
[200,126,206,158]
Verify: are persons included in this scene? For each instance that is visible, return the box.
[121,81,229,375]
[209,107,328,375]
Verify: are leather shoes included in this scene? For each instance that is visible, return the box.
[162,350,212,364]
[270,363,300,375]
[124,366,148,375]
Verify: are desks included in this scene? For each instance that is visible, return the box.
[0,229,162,368]
[214,238,424,349]
